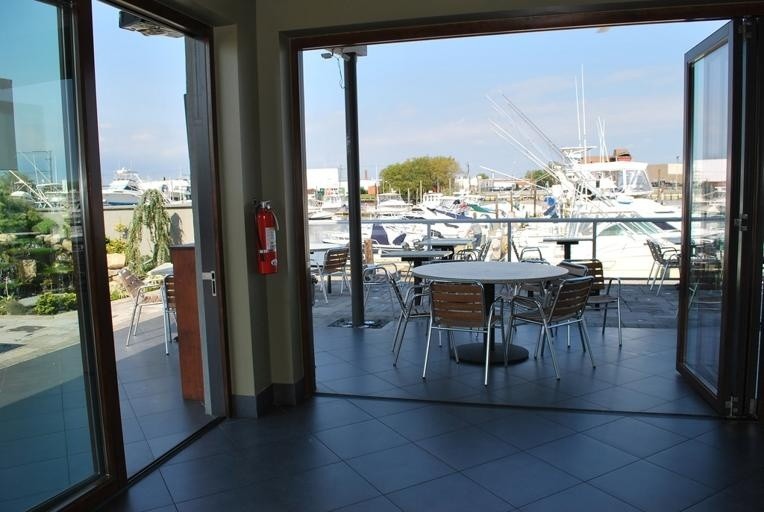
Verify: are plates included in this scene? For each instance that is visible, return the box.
[170,241,204,406]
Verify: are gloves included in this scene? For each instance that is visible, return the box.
[9,165,190,206]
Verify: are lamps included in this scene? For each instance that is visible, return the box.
[116,267,167,344]
[160,272,176,354]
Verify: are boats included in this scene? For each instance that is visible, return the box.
[307,65,725,285]
[9,165,190,206]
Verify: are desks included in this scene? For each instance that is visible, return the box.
[414,262,568,365]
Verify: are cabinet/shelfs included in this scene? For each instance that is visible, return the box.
[170,241,204,406]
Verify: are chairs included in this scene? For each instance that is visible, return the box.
[562,259,622,348]
[160,272,176,354]
[386,271,460,367]
[116,267,167,344]
[510,261,589,358]
[308,237,549,309]
[506,275,597,382]
[647,238,721,313]
[423,278,508,383]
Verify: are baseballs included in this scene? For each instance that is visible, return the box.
[254,200,278,274]
